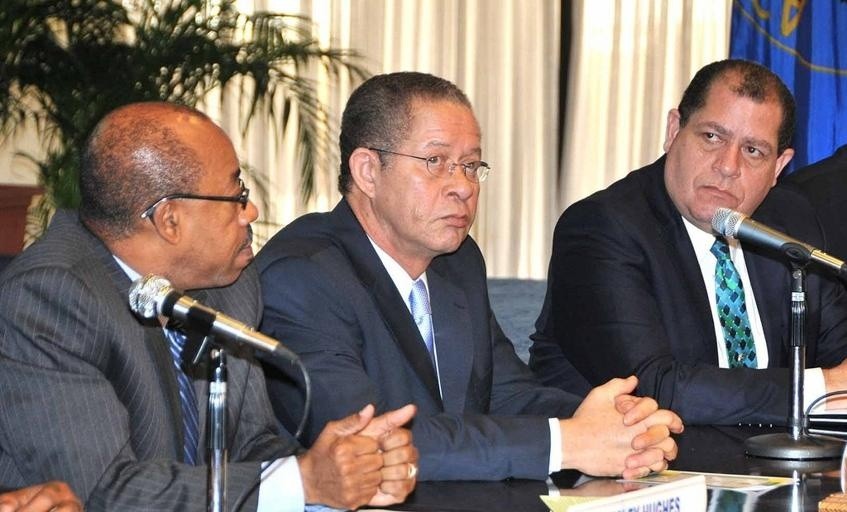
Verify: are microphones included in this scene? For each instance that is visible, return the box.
[711,206,847,277]
[128,272,299,366]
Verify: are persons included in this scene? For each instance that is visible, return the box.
[0,480,84,512]
[249,73,687,488]
[0,98,442,512]
[526,59,846,431]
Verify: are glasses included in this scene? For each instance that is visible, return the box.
[138,179,249,221]
[366,148,491,185]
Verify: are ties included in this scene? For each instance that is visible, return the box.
[708,235,761,370]
[165,325,200,467]
[407,279,436,379]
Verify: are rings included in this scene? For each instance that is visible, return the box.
[406,462,416,478]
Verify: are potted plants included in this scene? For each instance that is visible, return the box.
[0,1,131,254]
